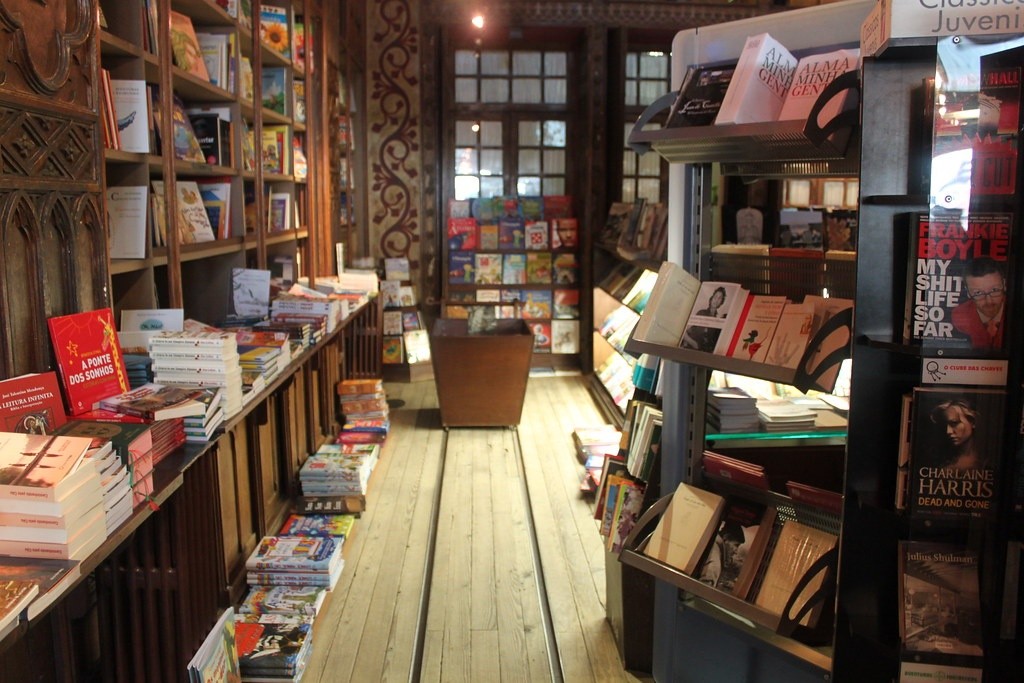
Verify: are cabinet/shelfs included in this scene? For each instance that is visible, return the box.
[2,2,1024,681]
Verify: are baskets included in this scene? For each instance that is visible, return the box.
[706,251,825,301]
[818,257,857,298]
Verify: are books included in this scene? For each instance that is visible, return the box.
[0,0,1022,683]
